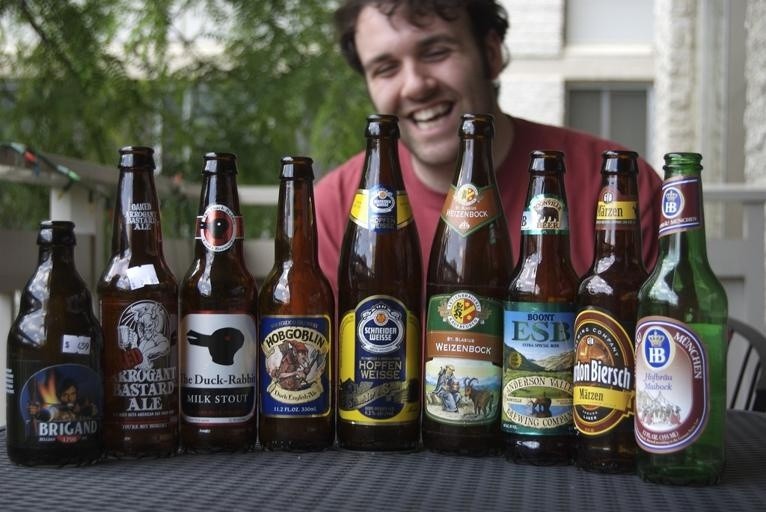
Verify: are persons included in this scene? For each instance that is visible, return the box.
[314,0,664,411]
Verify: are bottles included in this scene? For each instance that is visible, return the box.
[105,146,178,460]
[261,156,333,451]
[578,152,642,470]
[426,112,502,456]
[504,147,577,465]
[337,114,419,452]
[179,151,260,458]
[640,153,735,487]
[7,222,100,463]
